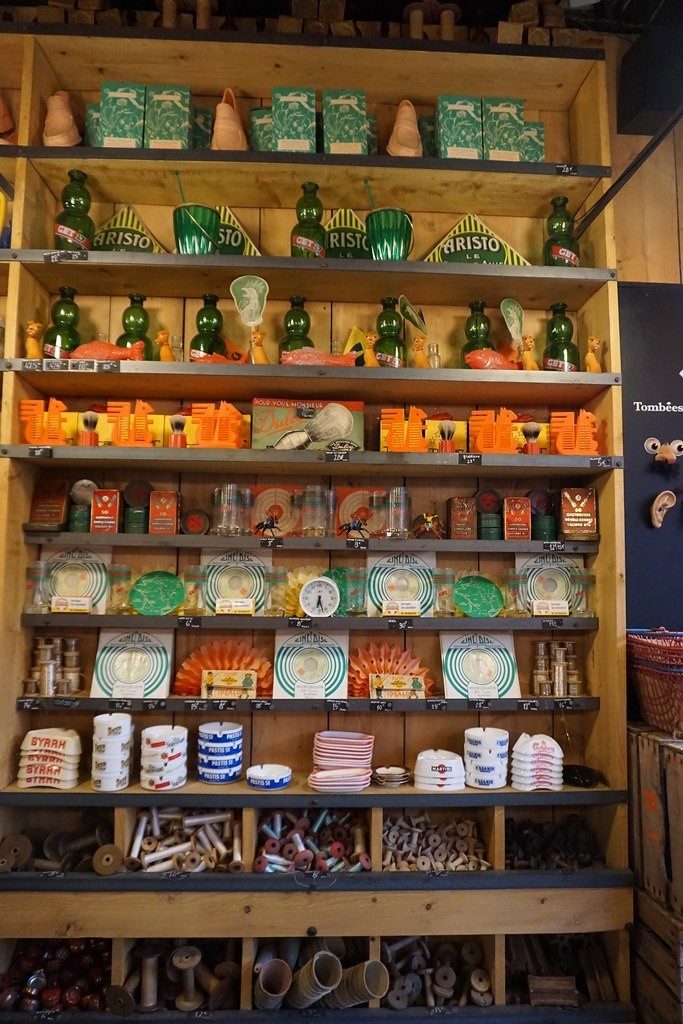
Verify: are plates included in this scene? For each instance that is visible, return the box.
[308,731,374,792]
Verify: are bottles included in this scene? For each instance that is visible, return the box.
[544,196,580,267]
[543,303,580,372]
[190,294,225,362]
[278,295,314,366]
[373,296,407,368]
[461,300,496,369]
[54,169,95,251]
[116,293,153,361]
[291,182,327,258]
[42,286,80,361]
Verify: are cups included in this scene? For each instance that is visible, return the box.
[24,560,51,614]
[184,564,206,616]
[325,490,338,538]
[370,486,412,539]
[241,488,253,537]
[346,567,368,617]
[173,204,220,255]
[503,567,527,616]
[217,484,241,537]
[365,207,415,261]
[289,492,303,537]
[107,564,131,615]
[432,568,455,617]
[302,487,325,537]
[210,488,221,536]
[570,568,595,617]
[264,565,287,616]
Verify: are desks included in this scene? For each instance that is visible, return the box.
[627,720,683,1024]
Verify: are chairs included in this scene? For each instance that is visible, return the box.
[250,87,379,155]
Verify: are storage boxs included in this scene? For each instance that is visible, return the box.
[419,95,545,163]
[19,412,251,449]
[252,398,365,452]
[84,80,213,150]
[379,420,577,455]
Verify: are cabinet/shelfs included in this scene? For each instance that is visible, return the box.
[0,25,637,1024]
